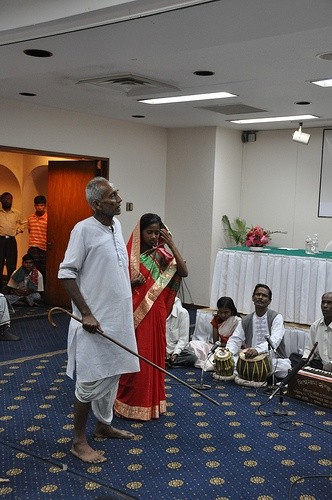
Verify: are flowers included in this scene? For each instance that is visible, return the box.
[244,223,270,247]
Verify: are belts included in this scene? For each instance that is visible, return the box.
[0,235,15,239]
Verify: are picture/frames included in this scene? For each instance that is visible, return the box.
[318,129,332,218]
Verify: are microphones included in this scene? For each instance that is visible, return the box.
[207,341,221,356]
[307,342,318,361]
[264,334,275,350]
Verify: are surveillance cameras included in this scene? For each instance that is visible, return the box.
[291,122,312,144]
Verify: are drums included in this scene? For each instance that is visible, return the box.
[214,349,234,376]
[236,348,271,383]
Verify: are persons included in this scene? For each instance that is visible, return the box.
[189,296,245,373]
[0,293,21,341]
[4,254,45,309]
[289,292,332,374]
[222,283,292,382]
[58,178,141,464]
[0,191,28,294]
[113,213,188,421]
[164,296,199,370]
[28,195,48,278]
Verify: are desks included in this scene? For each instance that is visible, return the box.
[209,246,332,327]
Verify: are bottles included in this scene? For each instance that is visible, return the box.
[305,233,319,255]
